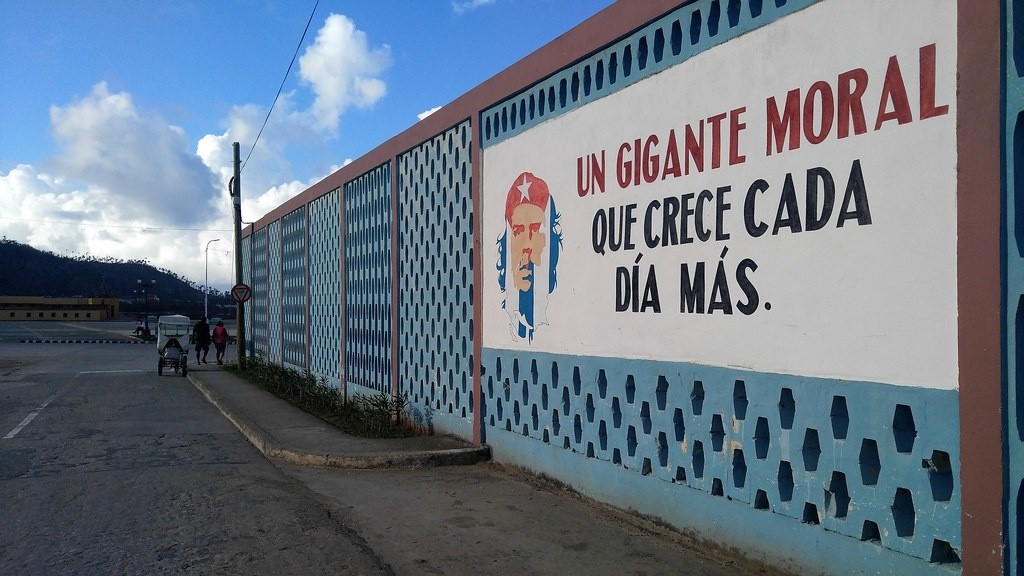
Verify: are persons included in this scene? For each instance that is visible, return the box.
[132,319,145,335]
[192,316,211,365]
[213,321,228,365]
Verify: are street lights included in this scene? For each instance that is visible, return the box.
[204,238,221,317]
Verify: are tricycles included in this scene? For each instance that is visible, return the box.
[154,316,190,376]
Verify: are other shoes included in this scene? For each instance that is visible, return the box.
[218,360,223,364]
[198,362,200,364]
[202,359,207,364]
